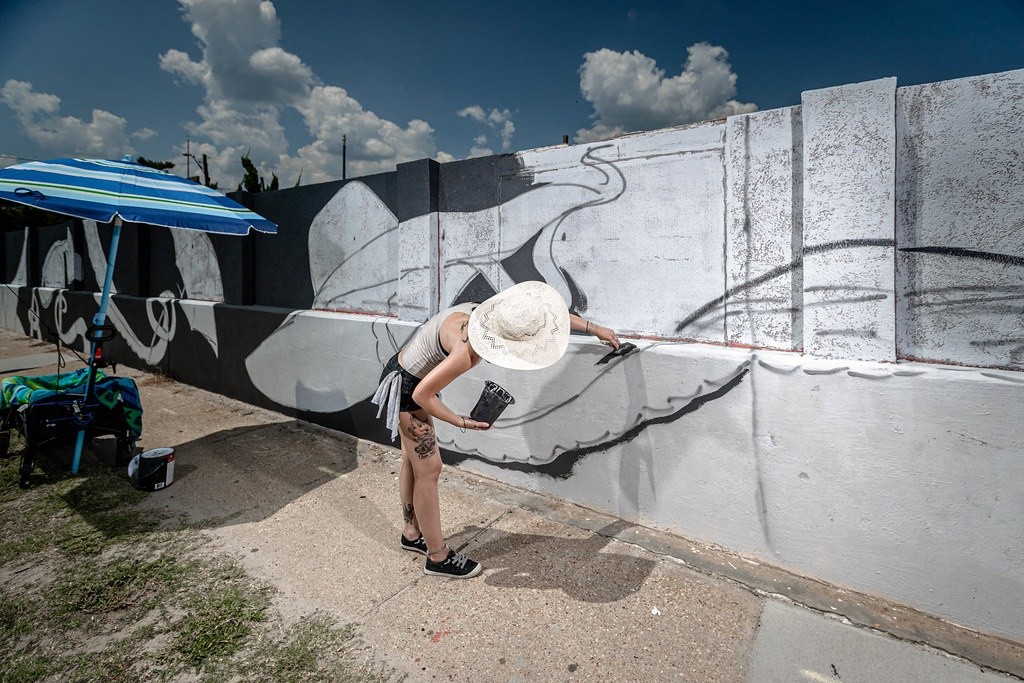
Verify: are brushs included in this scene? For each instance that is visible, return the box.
[600,339,640,358]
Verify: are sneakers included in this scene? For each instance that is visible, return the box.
[423,546,482,579]
[400,533,429,555]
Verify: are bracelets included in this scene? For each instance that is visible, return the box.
[586,319,593,336]
[460,414,466,433]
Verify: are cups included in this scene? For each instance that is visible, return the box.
[470,380,515,429]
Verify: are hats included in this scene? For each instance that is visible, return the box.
[467,281,571,370]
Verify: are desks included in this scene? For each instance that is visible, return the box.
[0,369,126,486]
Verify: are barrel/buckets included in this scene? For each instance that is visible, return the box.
[139,447,174,492]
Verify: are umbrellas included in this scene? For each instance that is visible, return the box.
[0,152,281,475]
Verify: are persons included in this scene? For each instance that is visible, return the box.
[372,281,621,579]
